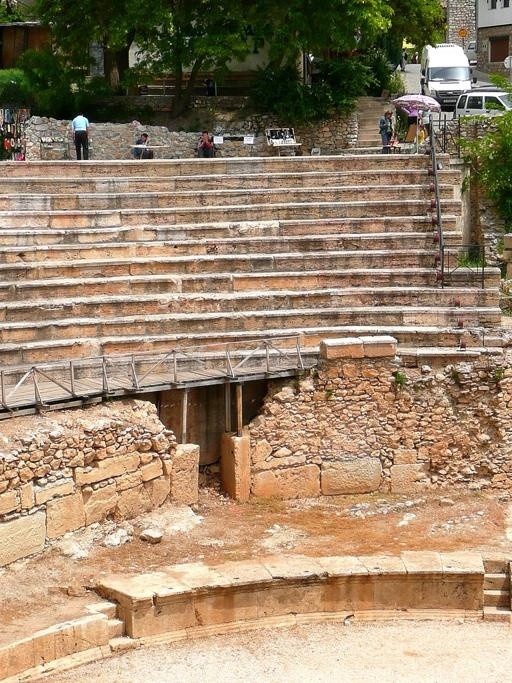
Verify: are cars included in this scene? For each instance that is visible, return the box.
[453,92,512,118]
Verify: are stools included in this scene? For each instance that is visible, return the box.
[383,146,391,154]
[393,146,401,154]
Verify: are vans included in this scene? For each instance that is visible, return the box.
[420,43,478,110]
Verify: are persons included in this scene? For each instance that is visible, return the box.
[196,129,215,158]
[411,51,419,63]
[378,109,395,153]
[133,132,154,160]
[406,107,419,125]
[70,111,92,159]
[418,108,431,137]
[399,47,407,71]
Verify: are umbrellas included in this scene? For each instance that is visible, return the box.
[392,93,441,153]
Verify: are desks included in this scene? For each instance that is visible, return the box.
[273,143,302,156]
[129,144,170,160]
